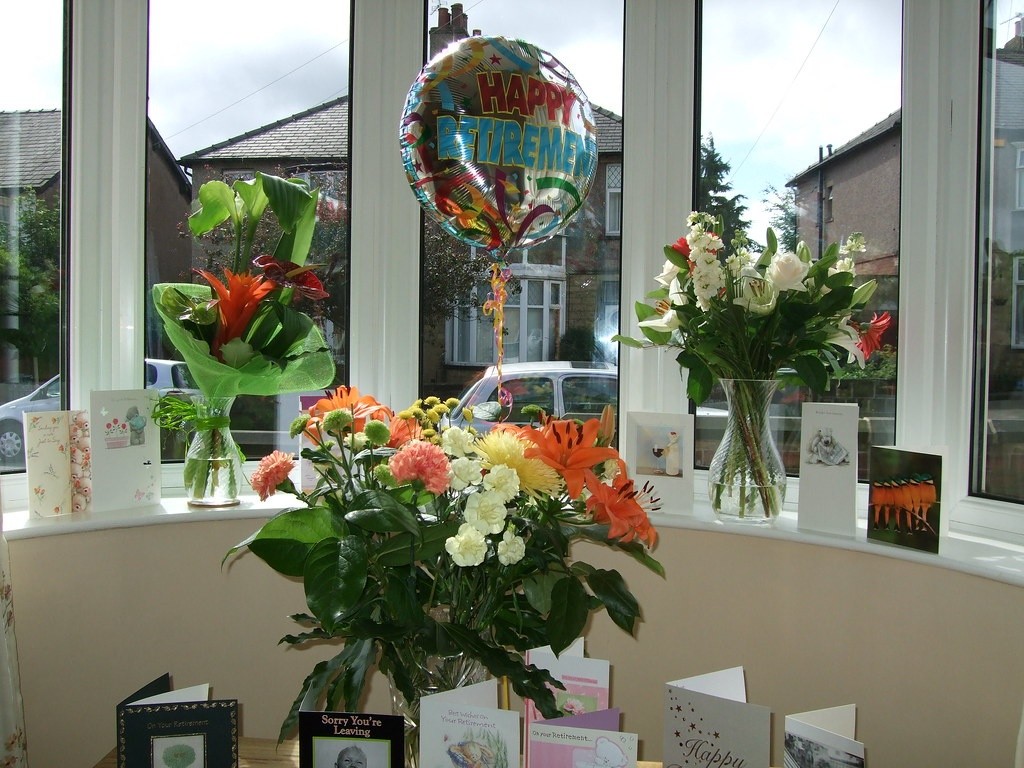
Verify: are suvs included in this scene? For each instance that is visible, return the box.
[0,355,205,466]
[438,359,624,451]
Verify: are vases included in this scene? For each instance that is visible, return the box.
[707,376,787,525]
[181,392,243,508]
[377,625,487,768]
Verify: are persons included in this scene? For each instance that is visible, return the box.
[333,745,369,768]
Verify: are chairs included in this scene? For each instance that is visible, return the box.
[590,393,616,414]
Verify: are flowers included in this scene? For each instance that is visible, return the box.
[632,209,894,405]
[253,370,657,709]
[135,169,333,372]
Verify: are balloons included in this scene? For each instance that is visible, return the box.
[398,33,601,284]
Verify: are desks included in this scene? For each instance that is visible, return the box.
[92,735,663,768]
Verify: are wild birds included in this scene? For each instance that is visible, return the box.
[871,480,883,531]
[919,473,936,531]
[882,479,893,530]
[910,473,921,530]
[890,478,900,532]
[900,477,914,536]
